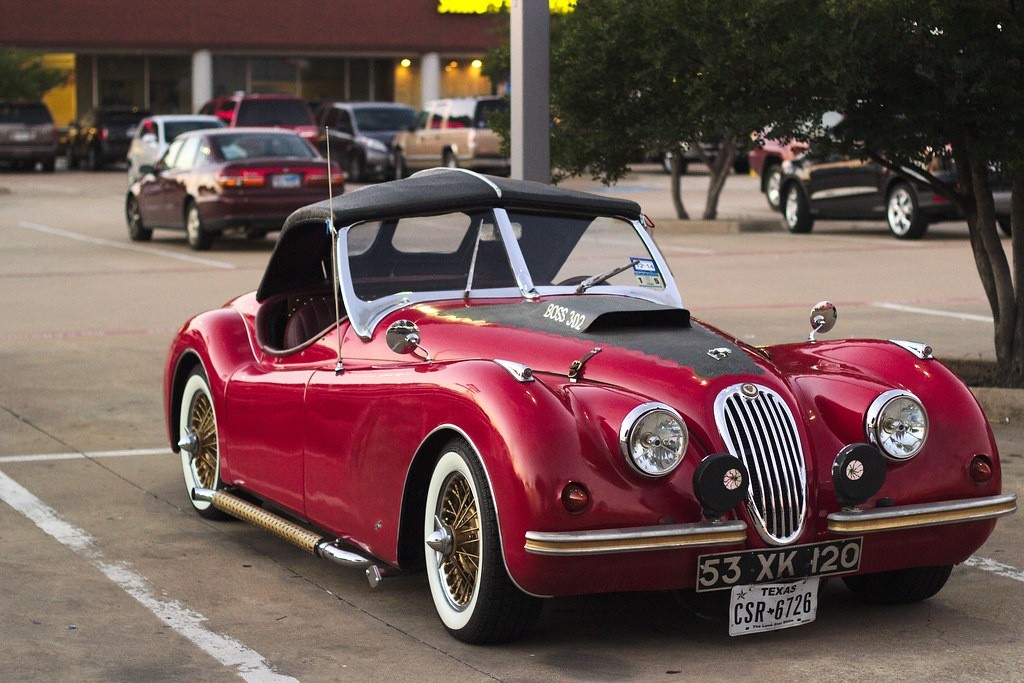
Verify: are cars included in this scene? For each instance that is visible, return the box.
[661,136,749,175]
[160,168,1022,651]
[126,113,245,192]
[750,124,829,210]
[124,127,347,251]
[316,101,425,183]
[65,106,154,171]
[776,148,1014,241]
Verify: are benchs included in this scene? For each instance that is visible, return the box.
[261,274,553,350]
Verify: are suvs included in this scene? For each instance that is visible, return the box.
[391,94,557,176]
[0,96,59,173]
[198,90,319,150]
